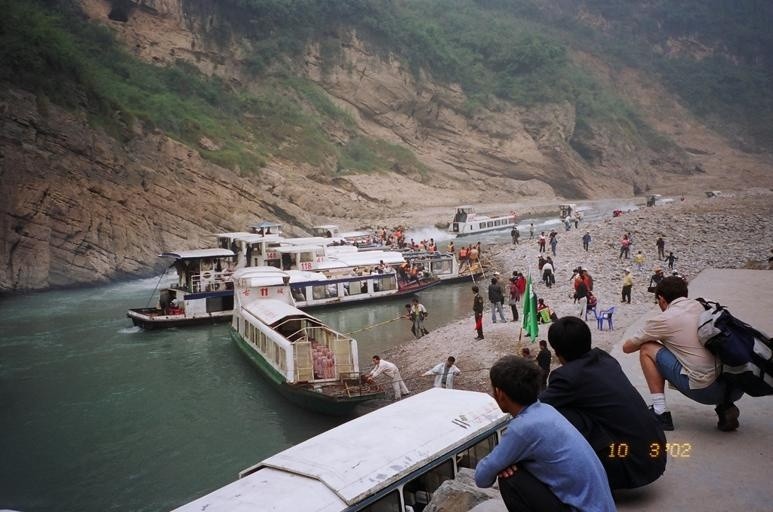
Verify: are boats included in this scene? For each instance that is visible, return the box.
[213,220,490,283]
[446,205,517,236]
[227,263,386,408]
[124,245,443,331]
[165,385,515,512]
[557,203,585,223]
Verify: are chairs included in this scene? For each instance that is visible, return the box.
[585,304,599,321]
[598,306,615,331]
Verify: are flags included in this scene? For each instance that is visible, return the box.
[520,273,538,341]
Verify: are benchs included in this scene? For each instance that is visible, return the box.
[364,455,479,512]
[290,333,334,378]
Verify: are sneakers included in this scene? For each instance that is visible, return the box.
[715,403,739,430]
[649,404,674,431]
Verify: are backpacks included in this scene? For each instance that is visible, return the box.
[694,298,773,397]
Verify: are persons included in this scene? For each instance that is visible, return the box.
[422,356,460,389]
[620,272,740,432]
[245,242,251,265]
[536,337,551,395]
[168,258,187,278]
[367,354,408,400]
[521,347,531,361]
[401,304,429,336]
[232,238,240,256]
[372,223,486,285]
[410,299,428,338]
[471,286,484,340]
[474,353,617,511]
[487,207,680,326]
[536,316,667,494]
